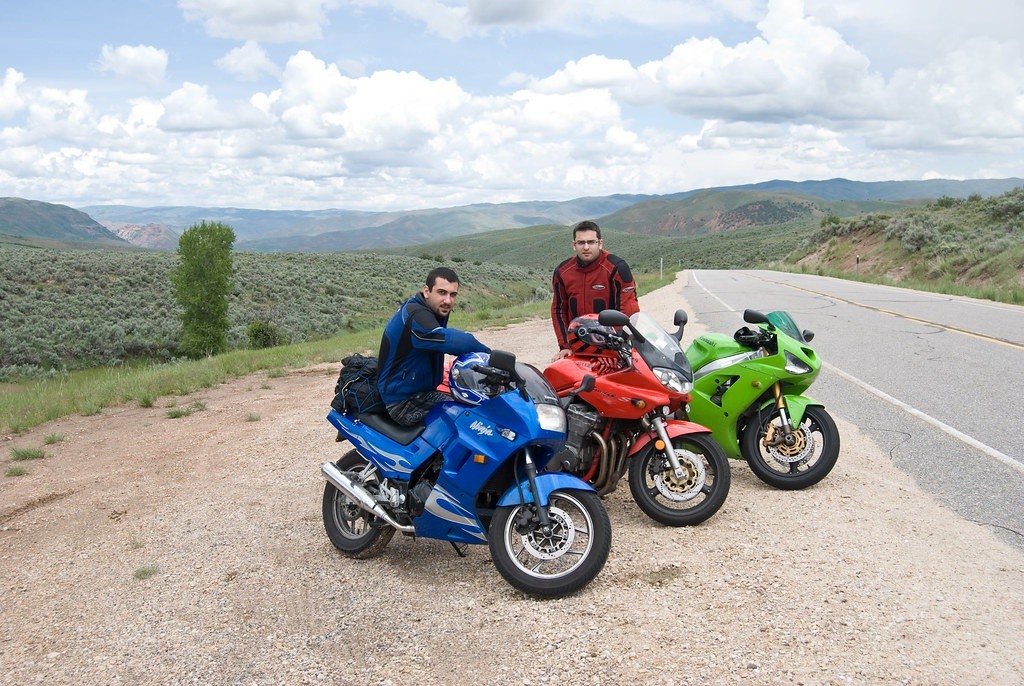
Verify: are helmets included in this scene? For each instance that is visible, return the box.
[449,352,504,405]
[568,313,619,358]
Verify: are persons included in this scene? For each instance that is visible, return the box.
[376,267,492,427]
[551,221,640,363]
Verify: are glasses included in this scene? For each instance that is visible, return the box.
[576,240,599,245]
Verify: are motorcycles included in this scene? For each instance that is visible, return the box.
[684,310,841,491]
[319,350,612,598]
[542,309,731,527]
